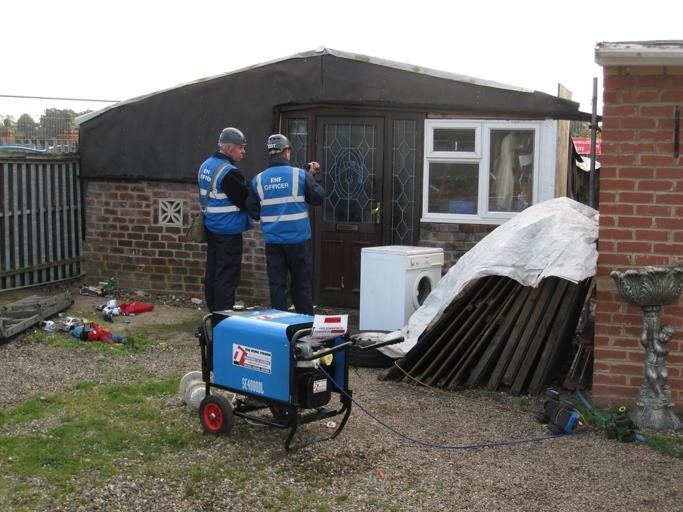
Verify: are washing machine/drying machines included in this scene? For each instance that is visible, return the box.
[357,244,444,331]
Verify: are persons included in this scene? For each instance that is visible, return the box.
[197,127,259,313]
[245,133,323,314]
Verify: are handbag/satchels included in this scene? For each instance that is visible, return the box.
[186,211,207,243]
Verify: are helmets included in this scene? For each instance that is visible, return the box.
[219,127,246,145]
[267,134,292,156]
